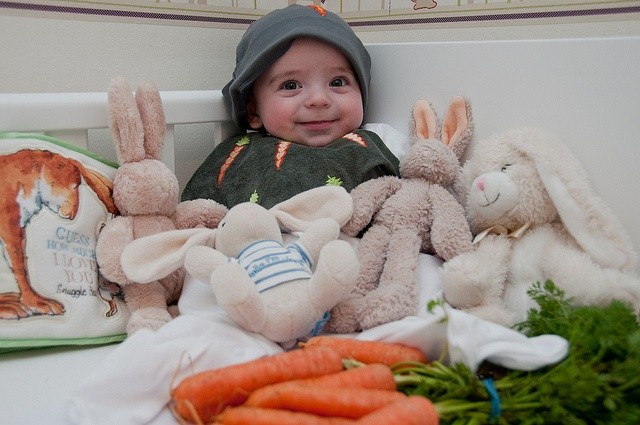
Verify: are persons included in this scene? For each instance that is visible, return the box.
[179,5,402,212]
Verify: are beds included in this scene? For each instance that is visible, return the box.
[2,91,640,425]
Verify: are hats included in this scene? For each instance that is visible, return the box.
[222,5,373,127]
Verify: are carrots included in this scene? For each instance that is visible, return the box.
[167,277,640,425]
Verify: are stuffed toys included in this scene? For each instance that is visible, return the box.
[94,82,230,340]
[124,185,362,343]
[441,126,635,329]
[318,97,476,336]
